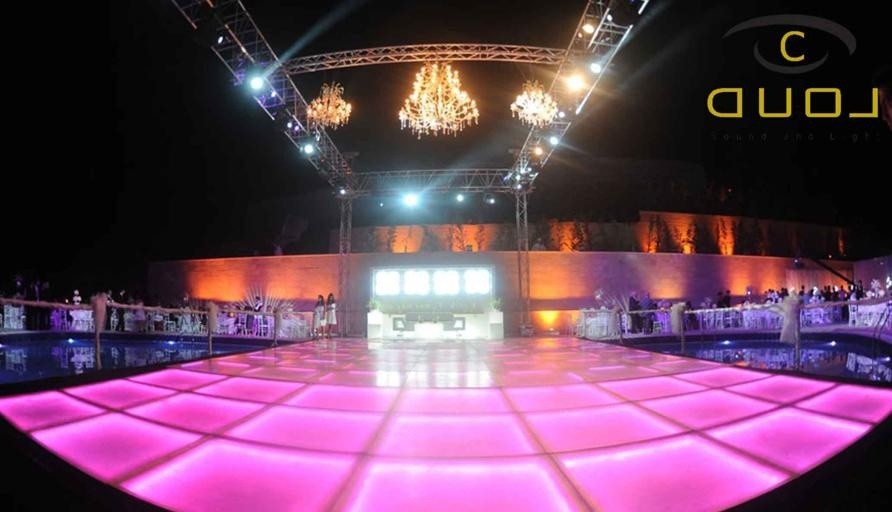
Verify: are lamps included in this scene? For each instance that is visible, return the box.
[399,62,480,140]
[307,81,352,130]
[510,80,560,129]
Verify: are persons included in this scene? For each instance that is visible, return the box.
[325,293,337,336]
[628,276,892,333]
[13,275,273,332]
[313,295,326,334]
